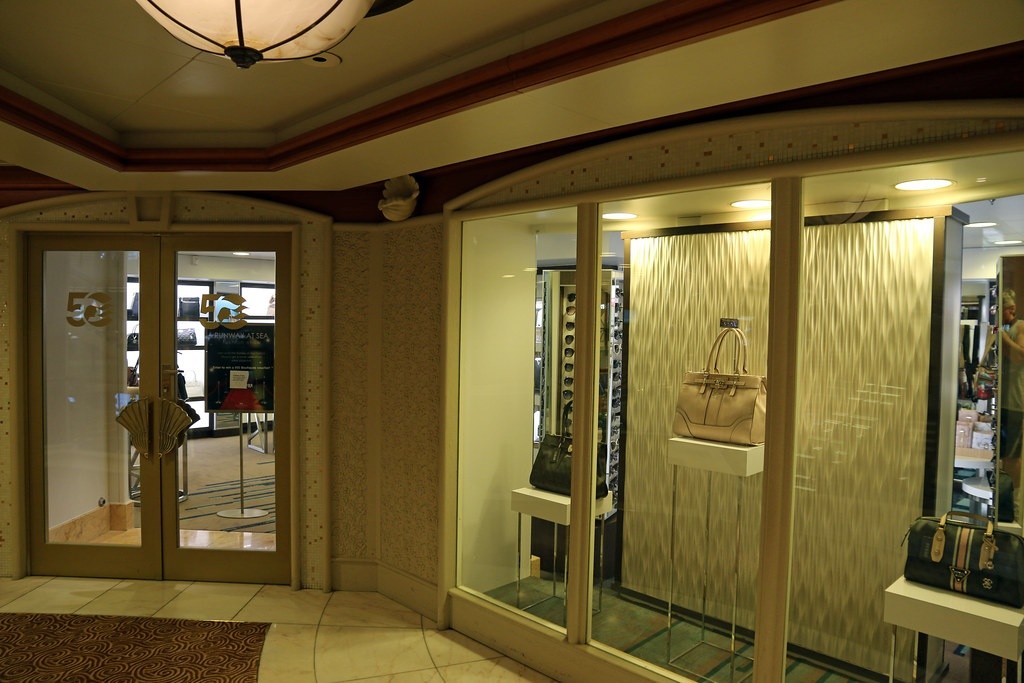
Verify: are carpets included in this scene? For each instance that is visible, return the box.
[0,611,273,683]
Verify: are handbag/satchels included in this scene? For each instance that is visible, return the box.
[127,359,140,387]
[131,292,138,317]
[997,470,1015,523]
[898,509,1024,610]
[179,296,200,317]
[128,323,138,345]
[177,370,189,401]
[671,327,768,447]
[529,399,609,500]
[177,328,197,346]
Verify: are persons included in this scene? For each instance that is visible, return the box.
[1001,290,1024,521]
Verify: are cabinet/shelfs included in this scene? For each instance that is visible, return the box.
[124,273,275,432]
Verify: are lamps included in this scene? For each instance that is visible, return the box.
[133,0,375,69]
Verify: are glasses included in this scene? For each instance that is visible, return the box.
[561,288,623,494]
